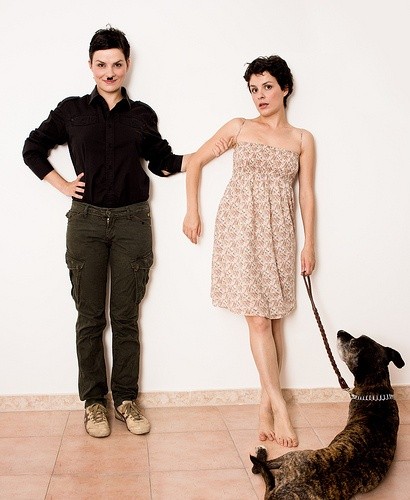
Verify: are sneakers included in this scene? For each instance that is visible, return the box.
[83,404,110,438]
[114,400,151,435]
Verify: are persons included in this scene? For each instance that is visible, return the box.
[23,30,235,438]
[184,56,315,447]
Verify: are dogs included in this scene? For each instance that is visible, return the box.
[249,330,405,500]
